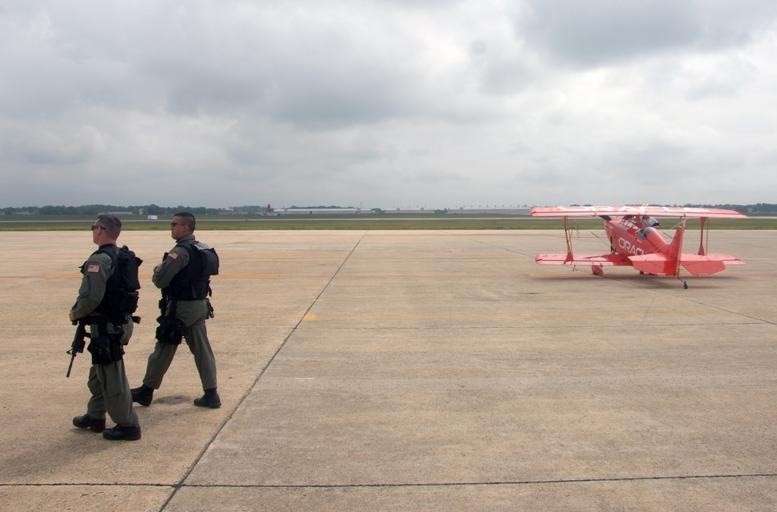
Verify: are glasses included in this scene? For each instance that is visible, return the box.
[90,226,107,231]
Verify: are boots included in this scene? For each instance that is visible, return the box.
[130,384,154,406]
[194,388,221,407]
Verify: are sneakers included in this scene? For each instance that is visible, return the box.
[103,424,141,440]
[73,413,105,432]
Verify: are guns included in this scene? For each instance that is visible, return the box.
[66,317,92,376]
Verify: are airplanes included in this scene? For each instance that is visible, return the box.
[528,203,751,289]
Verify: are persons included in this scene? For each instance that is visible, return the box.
[129,212,222,410]
[69,212,142,441]
